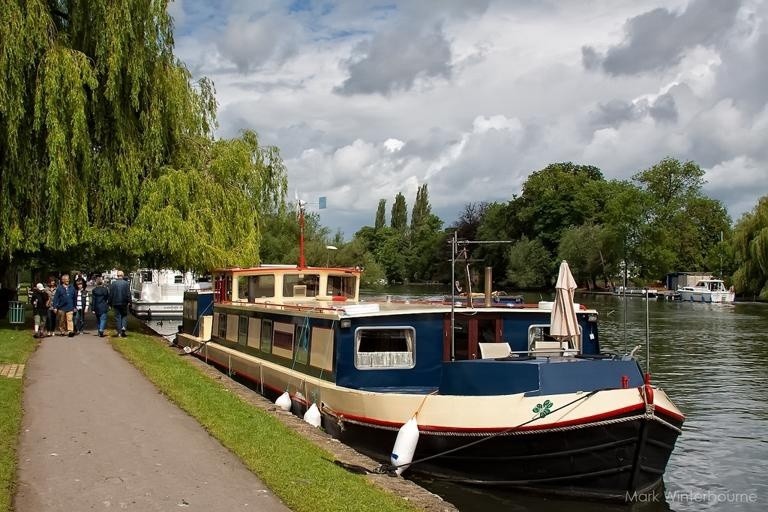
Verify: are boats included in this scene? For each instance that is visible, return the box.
[677,278,736,303]
[166,195,685,506]
[126,264,202,321]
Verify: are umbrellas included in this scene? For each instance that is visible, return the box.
[550,259,580,357]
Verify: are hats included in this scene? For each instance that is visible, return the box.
[36,283,45,291]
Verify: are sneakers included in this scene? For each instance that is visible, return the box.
[116,330,127,337]
[35,332,44,337]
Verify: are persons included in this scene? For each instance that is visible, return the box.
[91,277,111,337]
[31,272,88,338]
[108,270,131,336]
